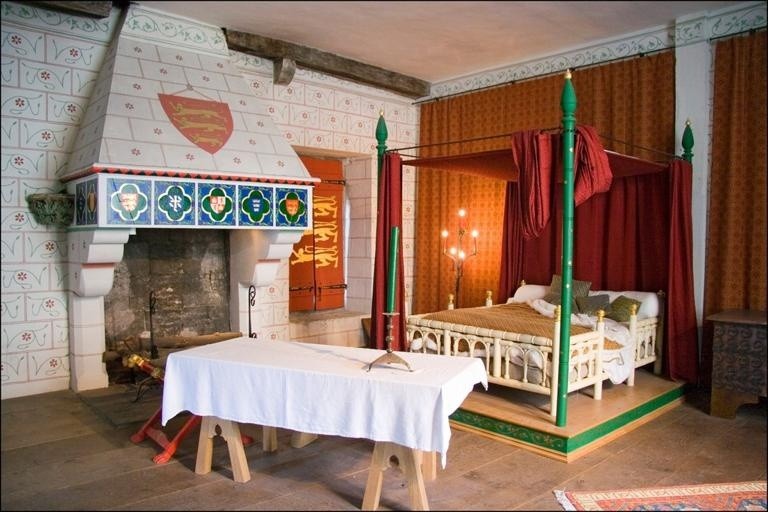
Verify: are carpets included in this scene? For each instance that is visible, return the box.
[555,480,768,512]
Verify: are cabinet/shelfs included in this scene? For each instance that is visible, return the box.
[710,310,768,418]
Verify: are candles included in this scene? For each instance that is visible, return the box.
[386,228,400,315]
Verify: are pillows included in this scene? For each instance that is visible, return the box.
[580,294,611,316]
[547,278,592,300]
[544,297,578,316]
[606,296,641,325]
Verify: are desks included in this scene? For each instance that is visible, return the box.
[168,337,480,512]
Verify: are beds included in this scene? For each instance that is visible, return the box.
[408,291,665,414]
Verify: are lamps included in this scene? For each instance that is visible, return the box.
[441,210,477,308]
